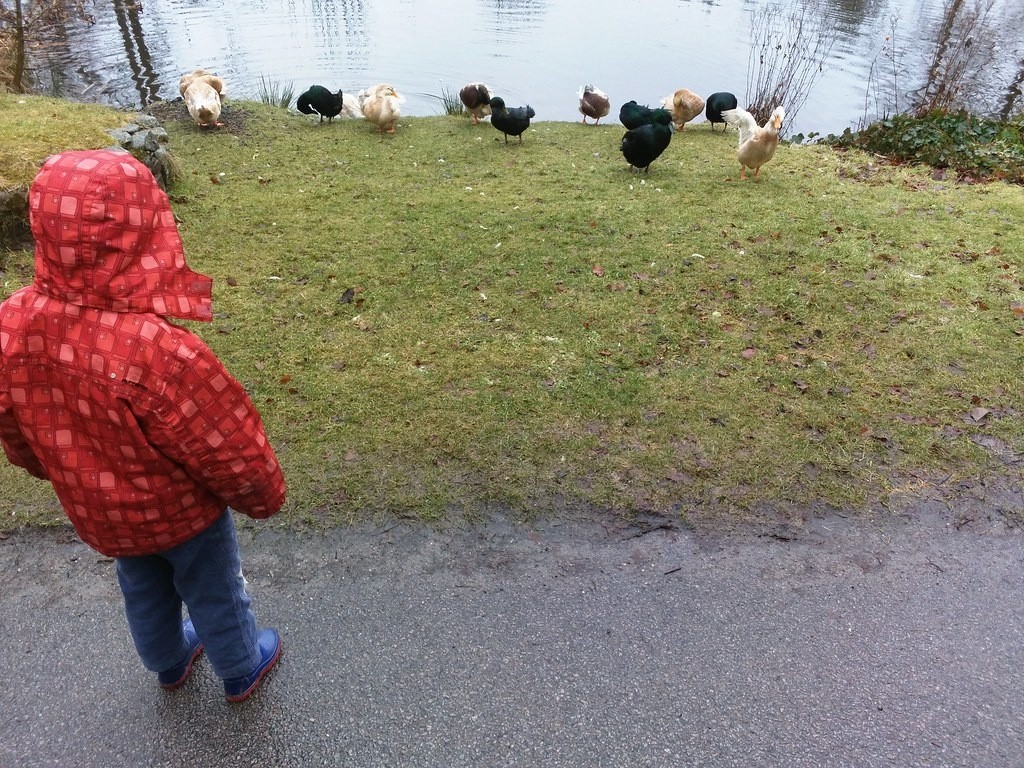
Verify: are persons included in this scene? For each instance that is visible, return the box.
[2,148,291,708]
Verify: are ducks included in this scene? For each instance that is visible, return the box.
[177,69,225,127]
[459,83,493,125]
[297,84,343,124]
[705,92,738,134]
[344,83,407,133]
[617,99,672,142]
[576,84,610,127]
[720,105,786,181]
[619,124,676,175]
[489,97,535,146]
[660,89,705,132]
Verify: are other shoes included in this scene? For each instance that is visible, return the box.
[158,618,202,687]
[223,629,282,703]
